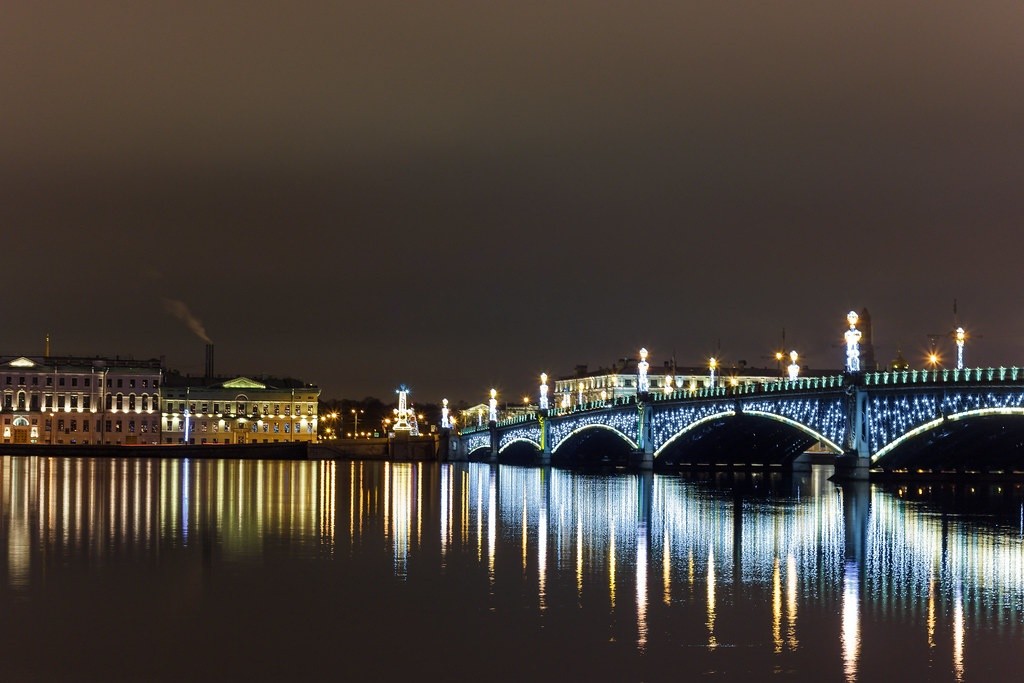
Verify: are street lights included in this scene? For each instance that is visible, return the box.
[351,409,364,437]
[843,310,863,371]
[709,358,716,388]
[637,347,650,392]
[524,397,529,414]
[788,350,799,381]
[393,384,412,431]
[441,398,449,428]
[578,383,584,404]
[478,409,483,426]
[539,372,549,409]
[956,327,965,369]
[489,388,497,421]
[182,410,191,442]
